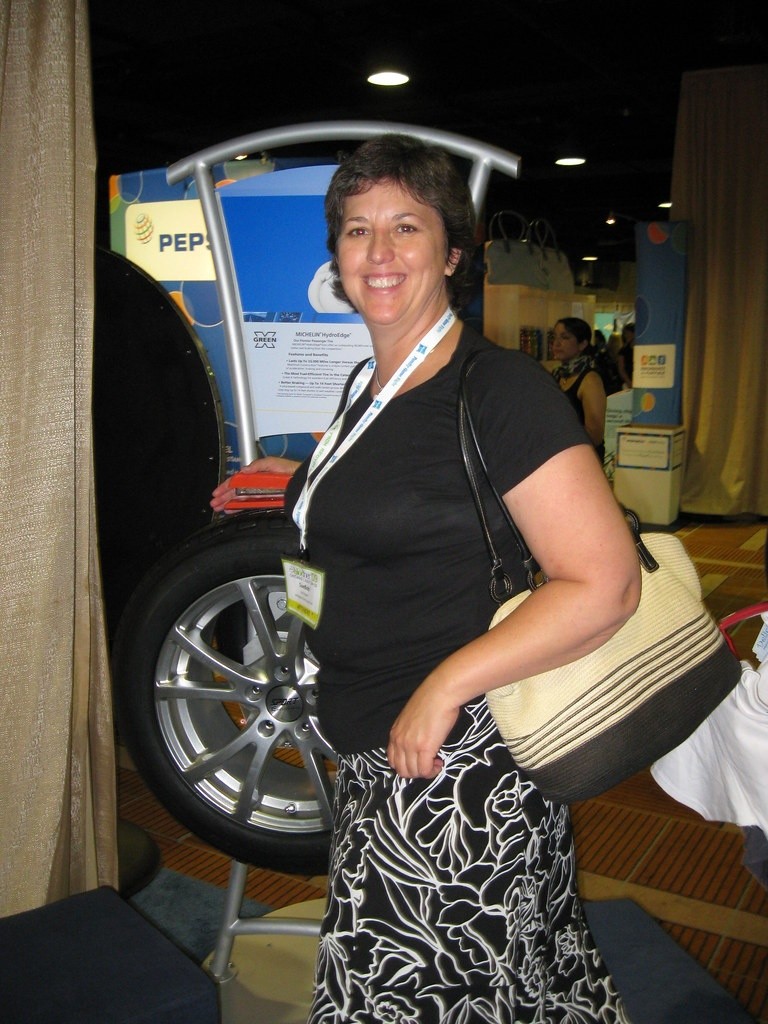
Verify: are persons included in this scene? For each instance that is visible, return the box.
[550,318,605,444]
[210,134,642,1023]
[595,323,634,395]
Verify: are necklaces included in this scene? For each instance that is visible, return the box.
[376,369,382,390]
[563,378,567,384]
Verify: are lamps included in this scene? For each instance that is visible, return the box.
[606,210,638,225]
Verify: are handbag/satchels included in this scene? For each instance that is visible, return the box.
[484,210,576,294]
[457,346,742,803]
